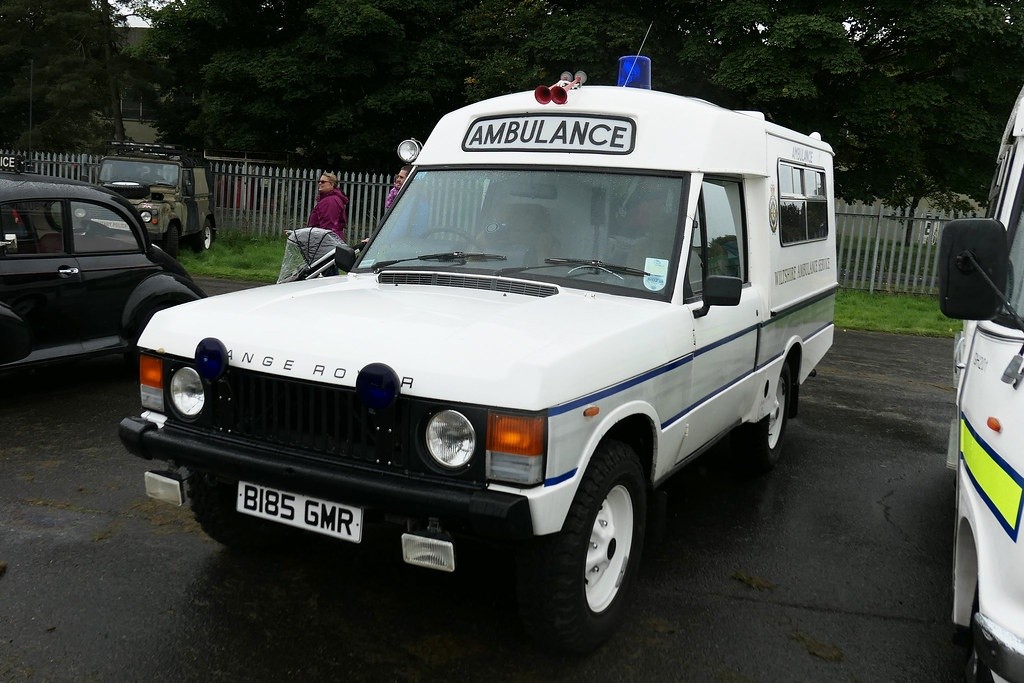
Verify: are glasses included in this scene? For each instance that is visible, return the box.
[317,179,328,184]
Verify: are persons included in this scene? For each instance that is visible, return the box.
[398,166,429,237]
[386,176,401,209]
[283,173,349,282]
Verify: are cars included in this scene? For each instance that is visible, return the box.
[0,172,207,376]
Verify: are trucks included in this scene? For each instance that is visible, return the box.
[937,86,1024,682]
[117,85,838,656]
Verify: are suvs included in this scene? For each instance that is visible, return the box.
[70,140,218,259]
